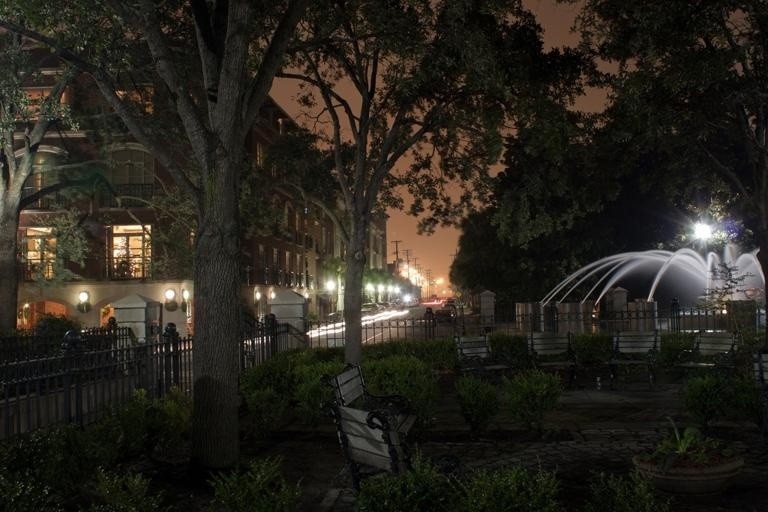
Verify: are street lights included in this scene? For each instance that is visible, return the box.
[326,280,400,322]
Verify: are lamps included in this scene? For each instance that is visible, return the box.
[164,288,189,312]
[77,292,90,313]
